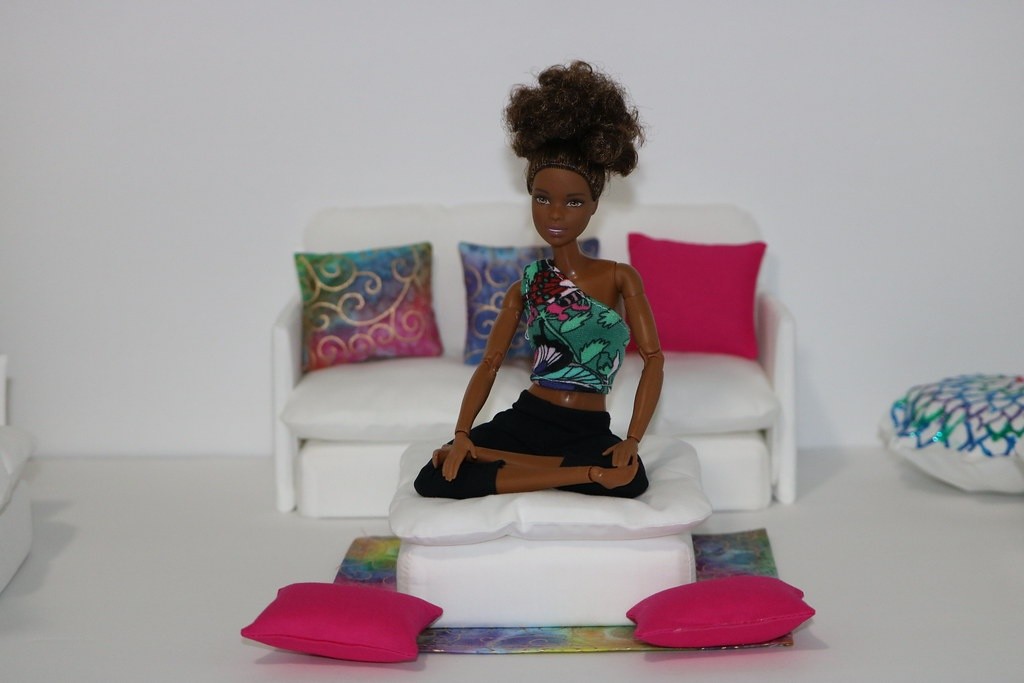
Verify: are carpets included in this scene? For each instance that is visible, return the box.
[322,524,797,657]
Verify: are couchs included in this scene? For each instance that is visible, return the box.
[267,205,805,512]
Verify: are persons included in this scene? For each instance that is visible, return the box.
[413,60,666,499]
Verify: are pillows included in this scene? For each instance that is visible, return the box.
[623,232,769,356]
[242,580,447,667]
[456,237,604,370]
[880,370,1024,499]
[284,234,447,372]
[624,570,820,649]
[385,436,708,548]
